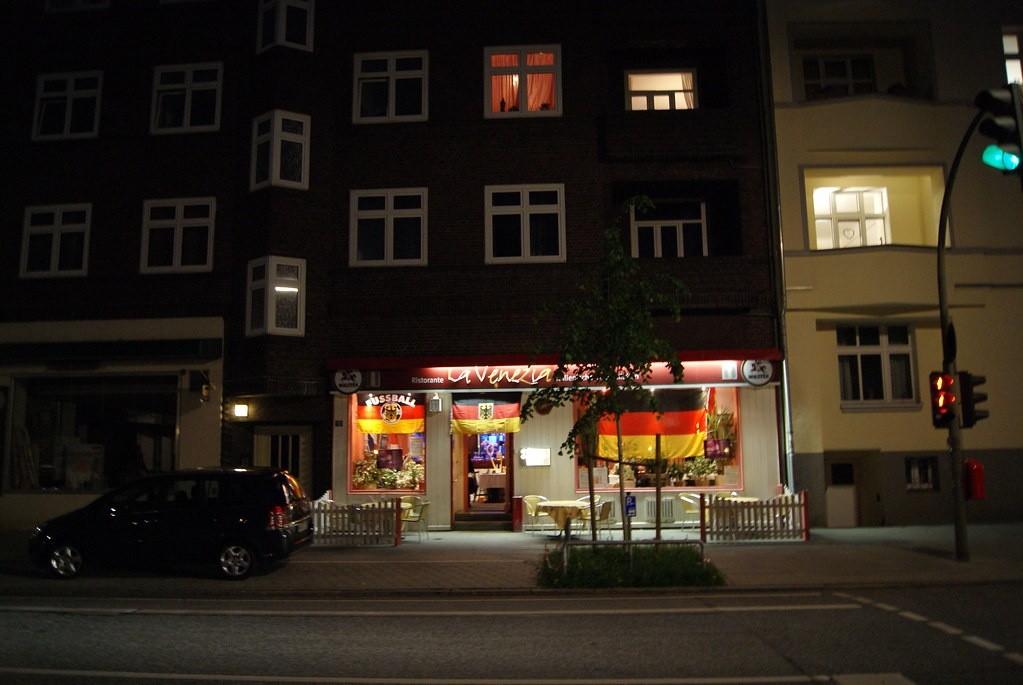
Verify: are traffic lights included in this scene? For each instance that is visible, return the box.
[928,372,958,430]
[960,368,991,430]
[978,81,1023,174]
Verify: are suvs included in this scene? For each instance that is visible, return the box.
[26,465,315,581]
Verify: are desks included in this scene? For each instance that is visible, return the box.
[534,501,594,541]
[713,496,761,528]
[362,502,413,537]
[477,474,506,501]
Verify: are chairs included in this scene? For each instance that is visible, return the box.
[523,495,557,539]
[574,494,602,535]
[677,492,741,535]
[763,494,793,526]
[577,499,614,539]
[321,498,357,534]
[173,485,206,504]
[393,496,430,542]
